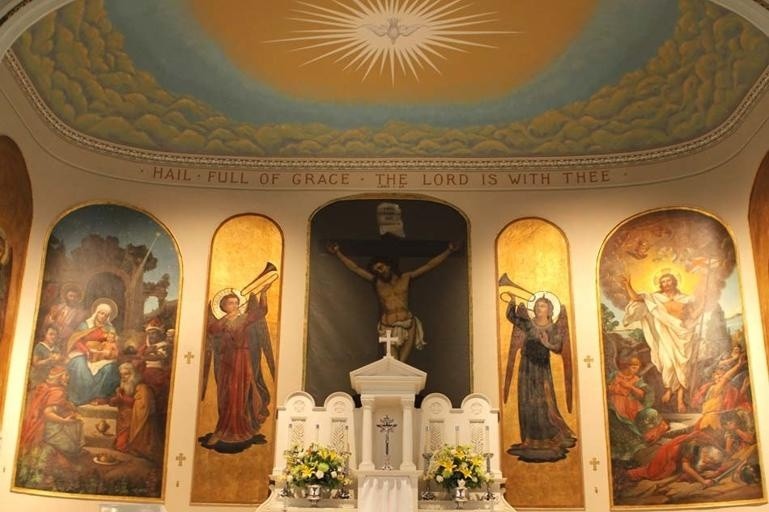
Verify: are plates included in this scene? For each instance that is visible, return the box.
[93,457,119,467]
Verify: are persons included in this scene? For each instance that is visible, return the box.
[196,282,277,441]
[324,238,460,364]
[503,289,578,452]
[17,277,177,500]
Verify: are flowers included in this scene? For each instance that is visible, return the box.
[423,444,490,488]
[287,446,343,490]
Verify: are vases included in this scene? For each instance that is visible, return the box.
[306,484,321,506]
[453,486,468,508]
